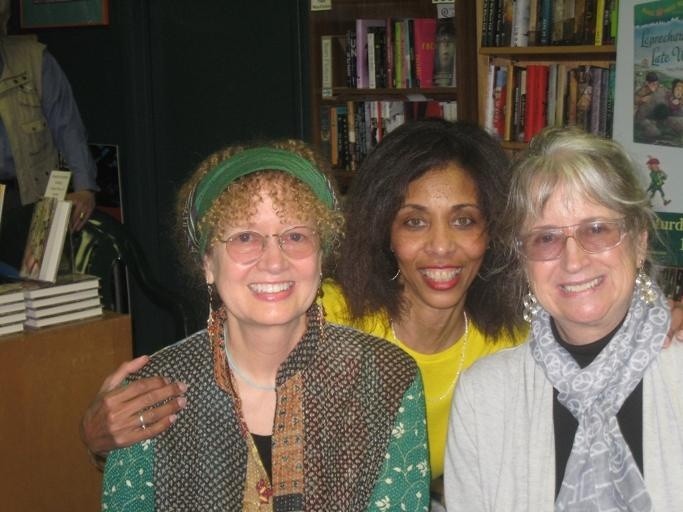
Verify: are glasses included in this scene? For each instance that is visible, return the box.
[211,225,329,263]
[513,216,637,262]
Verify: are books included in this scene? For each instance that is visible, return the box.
[319,101,458,171]
[481,1,618,48]
[1,269,106,338]
[17,195,72,284]
[485,56,616,142]
[322,19,457,98]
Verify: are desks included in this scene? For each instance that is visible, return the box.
[0,311,133,512]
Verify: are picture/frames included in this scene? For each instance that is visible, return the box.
[88,142,124,224]
[20,0,110,29]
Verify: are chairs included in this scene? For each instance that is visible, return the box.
[0,202,196,357]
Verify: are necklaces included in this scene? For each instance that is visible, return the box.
[390,308,469,404]
[222,323,275,390]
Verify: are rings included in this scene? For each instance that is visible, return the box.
[79,211,84,220]
[136,409,146,431]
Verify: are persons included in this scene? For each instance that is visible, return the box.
[97,137,433,511]
[82,117,682,512]
[442,128,682,512]
[1,34,101,271]
